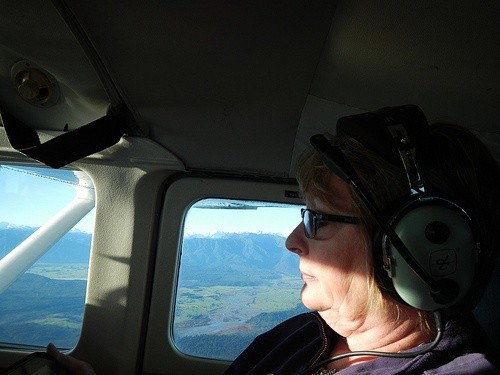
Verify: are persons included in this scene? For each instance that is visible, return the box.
[47,106,500,375]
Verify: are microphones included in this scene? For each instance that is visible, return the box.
[311,134,430,282]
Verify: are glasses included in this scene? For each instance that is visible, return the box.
[300,208,366,239]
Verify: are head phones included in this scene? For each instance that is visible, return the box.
[337,104,486,313]
